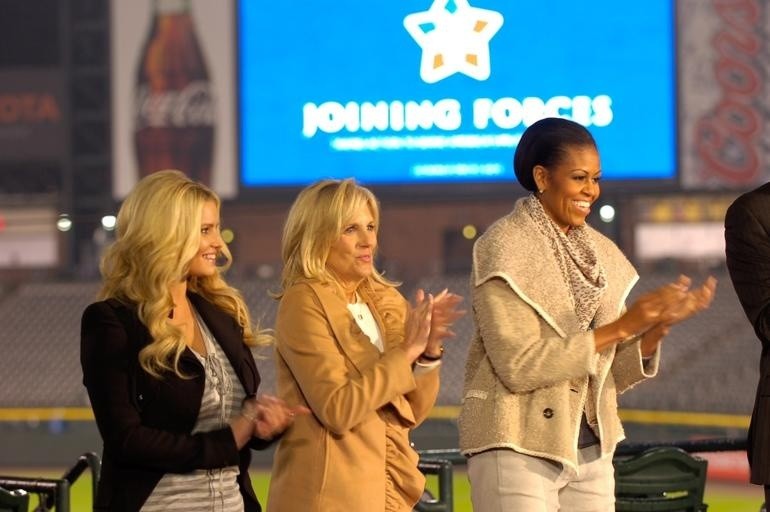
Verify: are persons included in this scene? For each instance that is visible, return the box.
[458,116,717,512]
[80,168,314,512]
[267,178,467,512]
[724,180,770,512]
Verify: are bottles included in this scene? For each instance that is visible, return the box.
[128,0,216,199]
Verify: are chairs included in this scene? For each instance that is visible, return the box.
[1,473,71,512]
[412,450,455,512]
[611,444,709,512]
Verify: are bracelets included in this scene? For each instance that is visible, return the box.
[239,408,263,425]
[420,346,443,362]
